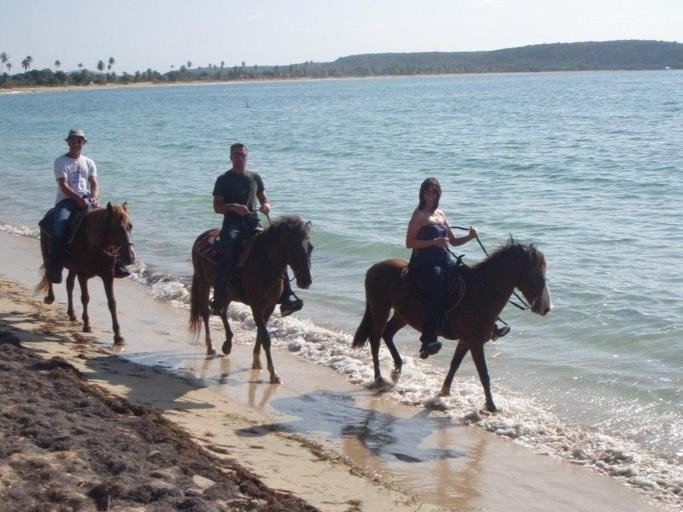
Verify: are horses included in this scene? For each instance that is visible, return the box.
[33,201,137,346]
[350,233,551,414]
[187,214,316,385]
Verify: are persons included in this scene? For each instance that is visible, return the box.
[209,143,303,319]
[404,177,511,359]
[48,128,131,284]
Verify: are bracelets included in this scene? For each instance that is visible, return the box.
[433,237,438,246]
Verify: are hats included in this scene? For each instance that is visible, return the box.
[63,128,88,146]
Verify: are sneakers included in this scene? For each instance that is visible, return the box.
[419,341,442,359]
[47,272,59,283]
[279,300,301,311]
[213,296,225,315]
[491,326,509,342]
[114,264,128,275]
